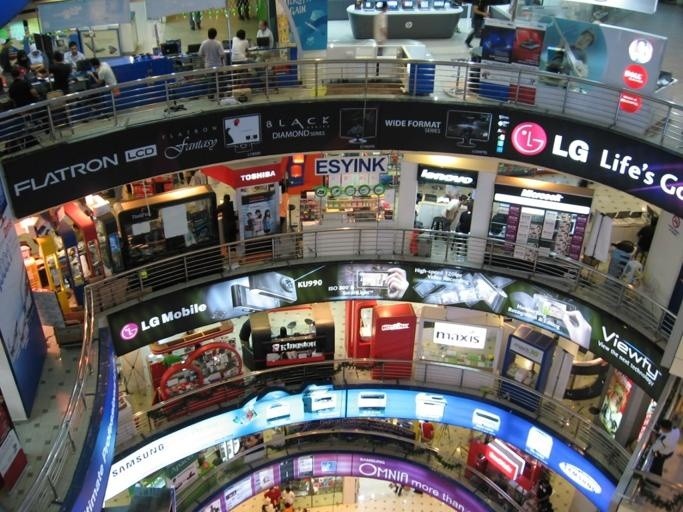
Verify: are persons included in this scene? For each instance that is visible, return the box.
[518,479,555,512]
[441,193,469,241]
[254,209,264,236]
[556,26,590,80]
[372,4,390,58]
[465,3,493,50]
[422,420,434,441]
[384,267,410,300]
[280,485,296,508]
[0,1,276,139]
[263,486,281,510]
[456,203,472,233]
[238,319,252,348]
[262,495,279,512]
[280,501,296,512]
[246,211,256,237]
[215,194,239,255]
[639,417,677,496]
[276,326,287,338]
[263,207,274,234]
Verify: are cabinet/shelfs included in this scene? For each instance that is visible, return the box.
[3,146,681,346]
[122,300,658,511]
[2,2,683,152]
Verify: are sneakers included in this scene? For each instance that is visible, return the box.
[464,40,473,49]
[208,95,215,99]
[220,94,224,98]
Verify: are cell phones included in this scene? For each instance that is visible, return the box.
[412,268,577,321]
[231,284,280,312]
[249,271,297,304]
[356,270,390,290]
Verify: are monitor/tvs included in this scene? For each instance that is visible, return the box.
[77,59,91,74]
[339,108,378,145]
[166,39,181,55]
[363,0,445,10]
[34,33,52,54]
[188,44,201,53]
[161,43,178,56]
[222,40,232,49]
[223,112,263,154]
[257,37,269,46]
[445,110,493,148]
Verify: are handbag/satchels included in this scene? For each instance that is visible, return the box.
[46,90,67,110]
[231,87,252,103]
